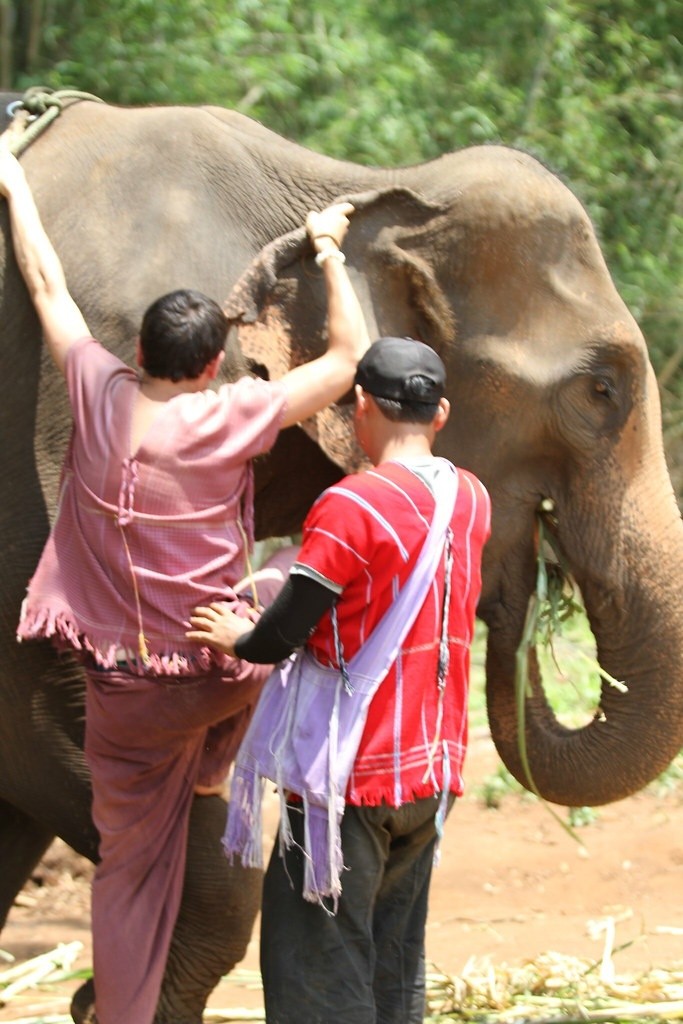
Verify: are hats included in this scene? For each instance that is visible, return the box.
[336,337,447,406]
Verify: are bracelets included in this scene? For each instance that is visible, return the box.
[315,250,347,267]
[312,234,341,247]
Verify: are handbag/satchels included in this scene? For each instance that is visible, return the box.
[218,648,374,916]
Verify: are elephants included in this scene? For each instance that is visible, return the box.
[1,89,682,1024]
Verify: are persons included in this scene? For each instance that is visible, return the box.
[0,147,369,1024]
[187,335,491,1023]
